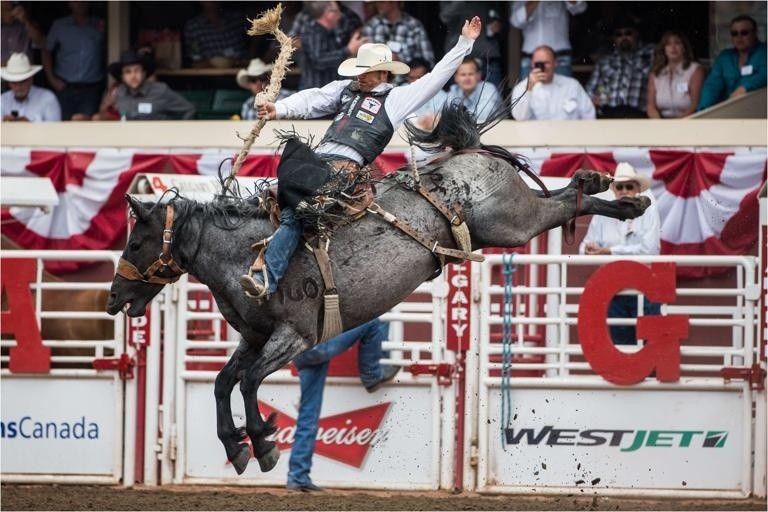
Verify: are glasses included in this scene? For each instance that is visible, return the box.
[247,75,268,83]
[613,30,632,38]
[614,184,633,191]
[729,29,750,36]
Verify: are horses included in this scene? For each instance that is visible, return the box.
[104,51,651,475]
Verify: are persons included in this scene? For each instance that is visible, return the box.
[110,50,196,120]
[577,162,659,346]
[41,1,107,121]
[444,58,508,122]
[648,31,702,120]
[184,0,245,70]
[362,2,435,83]
[1,2,43,66]
[509,1,588,77]
[1,53,62,122]
[235,57,301,122]
[697,15,768,113]
[587,19,657,118]
[282,316,401,494]
[440,0,505,82]
[287,2,370,88]
[240,13,482,303]
[395,57,447,129]
[509,45,595,119]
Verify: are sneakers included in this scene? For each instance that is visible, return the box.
[241,273,266,299]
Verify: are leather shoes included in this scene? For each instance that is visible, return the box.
[365,364,399,392]
[286,482,326,495]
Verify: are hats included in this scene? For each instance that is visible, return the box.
[608,162,649,192]
[107,51,156,81]
[337,42,411,79]
[236,57,272,88]
[0,52,42,83]
